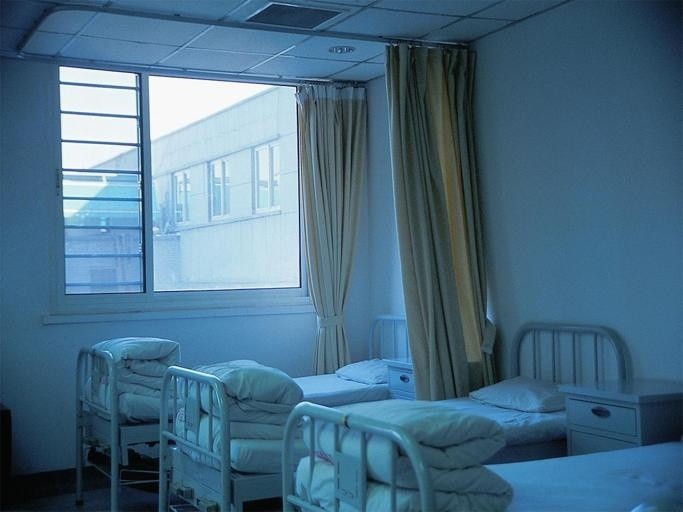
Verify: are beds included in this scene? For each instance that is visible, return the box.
[73,314,682,512]
